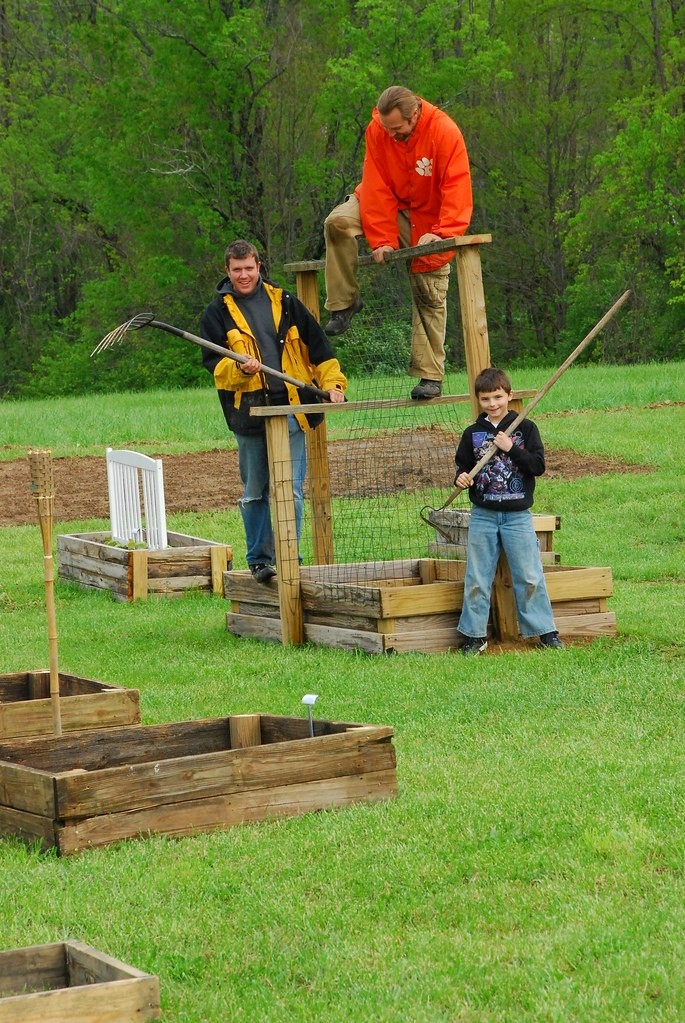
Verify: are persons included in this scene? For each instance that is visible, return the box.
[201,240,348,582]
[324,86,474,399]
[454,368,564,655]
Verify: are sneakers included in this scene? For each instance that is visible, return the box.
[462,637,488,658]
[411,378,442,398]
[539,633,564,650]
[249,564,278,583]
[325,299,363,335]
